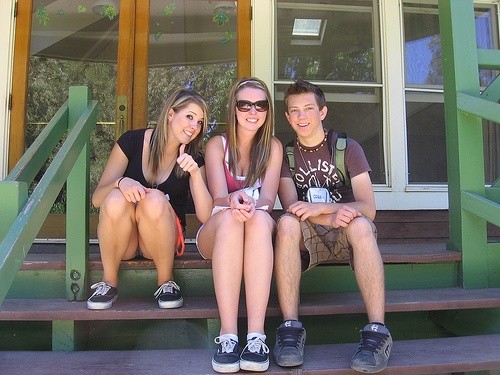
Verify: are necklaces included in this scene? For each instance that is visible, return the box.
[298,132,335,188]
[297,128,328,152]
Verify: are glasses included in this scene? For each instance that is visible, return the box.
[234,100,270,112]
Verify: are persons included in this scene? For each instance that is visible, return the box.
[87,90,214,309]
[274,80,393,374]
[196,78,283,373]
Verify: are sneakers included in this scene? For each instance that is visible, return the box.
[212,338,240,373]
[351,323,393,373]
[272,320,306,367]
[240,337,270,372]
[87,282,119,309]
[157,281,183,308]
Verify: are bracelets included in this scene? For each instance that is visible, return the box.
[118,177,126,188]
